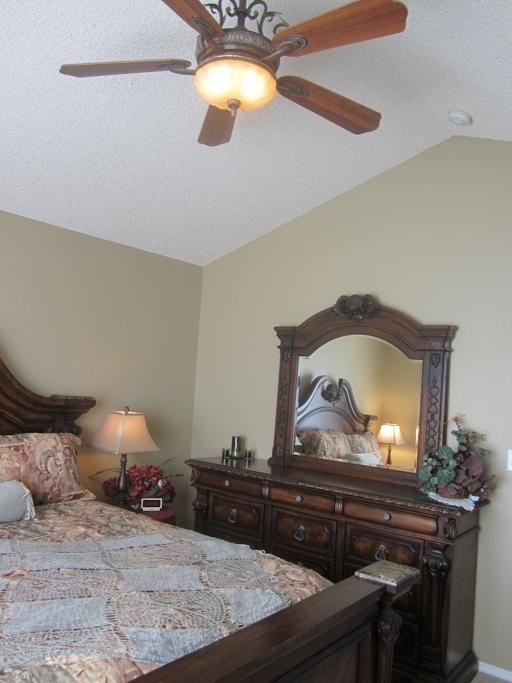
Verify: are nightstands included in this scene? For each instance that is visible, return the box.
[133,503,177,526]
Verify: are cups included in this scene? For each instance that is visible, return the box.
[231,435,240,457]
[222,448,230,458]
[244,448,252,458]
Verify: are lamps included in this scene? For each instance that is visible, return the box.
[87,406,160,513]
[376,423,404,465]
[192,51,278,119]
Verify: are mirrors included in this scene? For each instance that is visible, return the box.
[267,293,459,491]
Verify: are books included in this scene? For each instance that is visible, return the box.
[355,559,421,586]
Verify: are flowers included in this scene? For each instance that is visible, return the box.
[89,455,184,512]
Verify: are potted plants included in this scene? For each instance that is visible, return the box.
[417,409,499,501]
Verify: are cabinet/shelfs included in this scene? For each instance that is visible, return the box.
[184,455,480,682]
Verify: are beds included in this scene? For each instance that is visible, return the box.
[0,354,423,683]
[291,373,417,474]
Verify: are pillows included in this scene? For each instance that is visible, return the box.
[0,431,97,524]
[296,428,382,467]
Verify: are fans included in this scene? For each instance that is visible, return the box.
[60,1,409,148]
